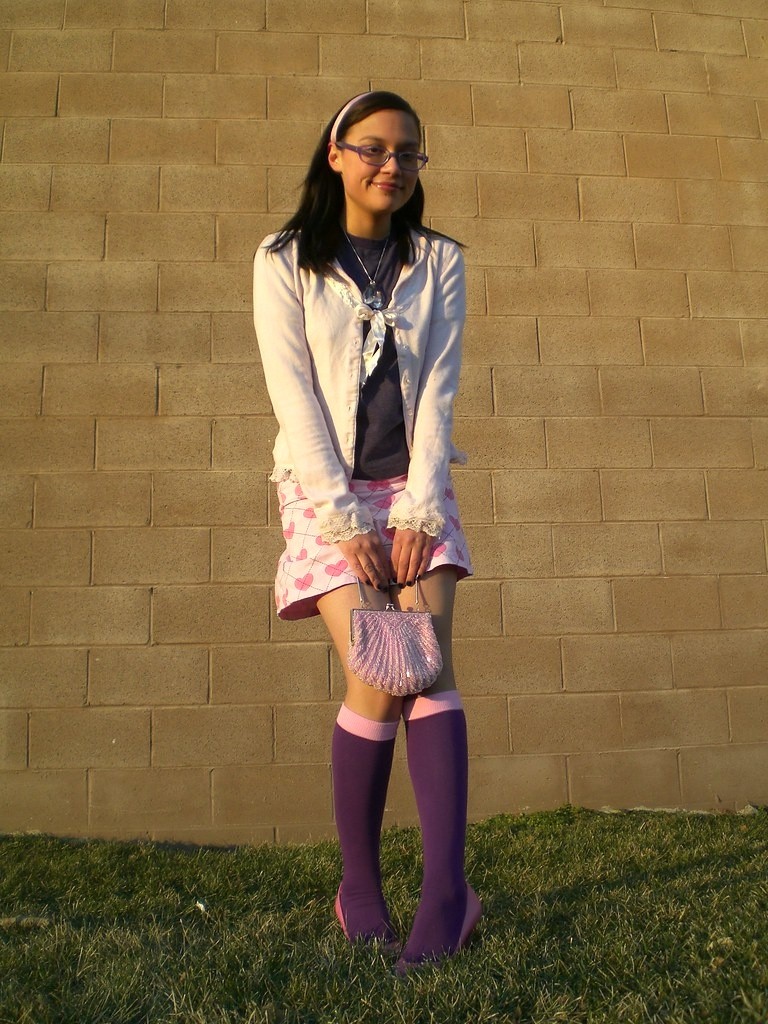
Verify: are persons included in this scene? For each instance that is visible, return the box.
[253,91,481,980]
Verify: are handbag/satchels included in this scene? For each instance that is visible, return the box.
[349,576,443,696]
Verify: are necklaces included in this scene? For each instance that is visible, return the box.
[342,224,392,308]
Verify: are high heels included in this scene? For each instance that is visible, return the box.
[335,882,402,952]
[394,880,482,970]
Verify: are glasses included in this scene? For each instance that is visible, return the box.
[335,141,429,172]
